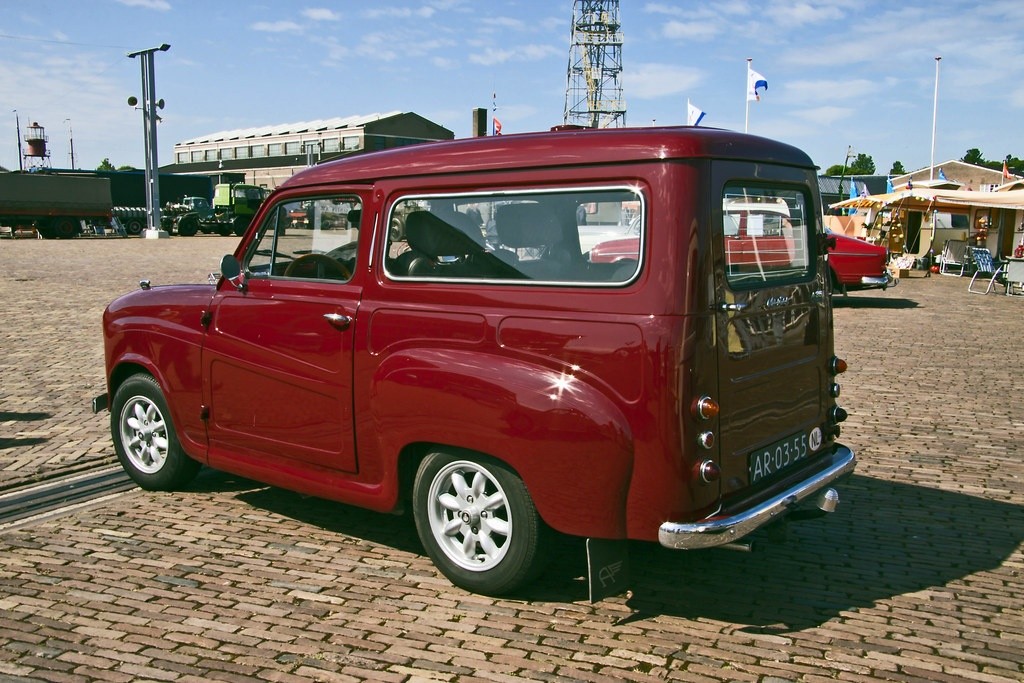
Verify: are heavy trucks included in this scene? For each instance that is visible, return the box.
[0,172,275,237]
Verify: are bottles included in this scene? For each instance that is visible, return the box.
[1015,245,1023,258]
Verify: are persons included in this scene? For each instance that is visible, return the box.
[465,198,481,221]
[577,203,588,224]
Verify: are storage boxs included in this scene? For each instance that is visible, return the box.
[888,267,910,278]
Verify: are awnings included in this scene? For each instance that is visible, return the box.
[828,187,1024,214]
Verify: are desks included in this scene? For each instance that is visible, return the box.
[1004,256,1024,296]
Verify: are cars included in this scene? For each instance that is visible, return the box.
[91,124,857,599]
[580,203,899,300]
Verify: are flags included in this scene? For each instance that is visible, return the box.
[848,181,859,217]
[688,104,706,126]
[887,179,895,194]
[491,117,504,132]
[939,168,947,181]
[747,69,768,102]
[1003,162,1012,180]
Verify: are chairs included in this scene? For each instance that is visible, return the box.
[938,238,968,277]
[496,204,564,281]
[967,247,1009,295]
[405,210,495,277]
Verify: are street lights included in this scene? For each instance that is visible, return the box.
[930,55,943,183]
[12,109,24,172]
